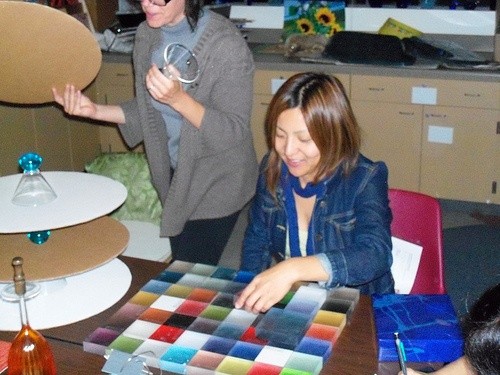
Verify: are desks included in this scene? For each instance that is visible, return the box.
[0,255,378,375]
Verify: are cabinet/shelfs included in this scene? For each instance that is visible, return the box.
[0,61,500,205]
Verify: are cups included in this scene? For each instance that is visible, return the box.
[143,41,202,97]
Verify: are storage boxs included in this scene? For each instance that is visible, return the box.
[370,290,465,363]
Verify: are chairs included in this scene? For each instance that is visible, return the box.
[387,188,446,295]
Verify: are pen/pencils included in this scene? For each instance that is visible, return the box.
[393,332,407,375]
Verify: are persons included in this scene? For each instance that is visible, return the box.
[234,72,394,314]
[51,0,259,267]
[398,283,500,375]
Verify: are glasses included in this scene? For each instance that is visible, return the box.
[141,0,174,6]
